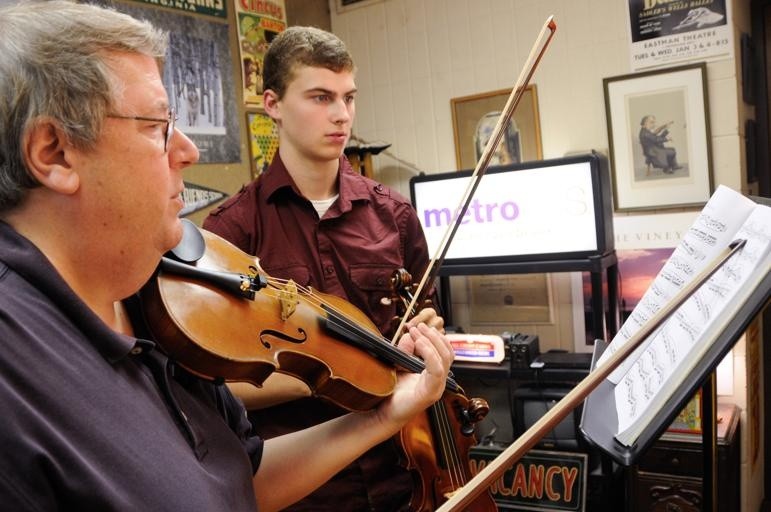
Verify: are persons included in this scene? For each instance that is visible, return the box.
[198,25,447,510]
[636,113,684,177]
[0,1,458,510]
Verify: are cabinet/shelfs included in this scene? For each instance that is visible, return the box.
[426,253,743,510]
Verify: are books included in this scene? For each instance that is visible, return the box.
[594,181,771,447]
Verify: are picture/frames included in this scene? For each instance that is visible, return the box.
[244,107,279,185]
[602,61,714,215]
[463,272,559,329]
[334,0,383,13]
[449,84,543,173]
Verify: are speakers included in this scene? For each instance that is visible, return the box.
[510,334,540,377]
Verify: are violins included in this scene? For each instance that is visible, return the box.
[381,270,498,512]
[121,221,491,436]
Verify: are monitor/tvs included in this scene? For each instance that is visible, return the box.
[514,386,580,451]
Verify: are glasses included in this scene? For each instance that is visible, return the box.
[103,107,177,153]
